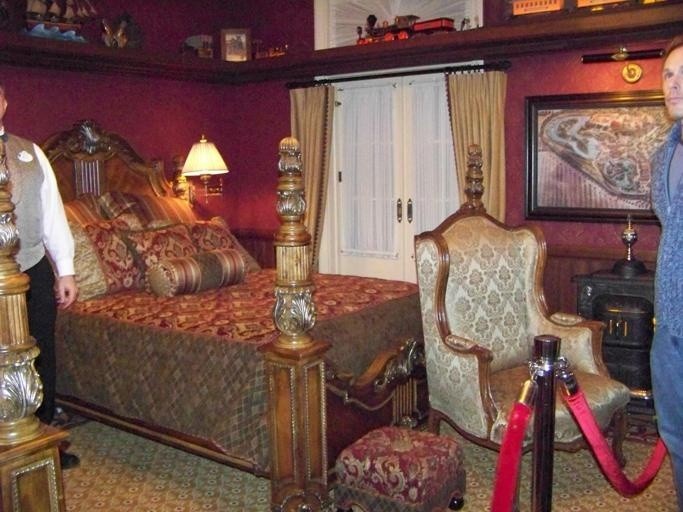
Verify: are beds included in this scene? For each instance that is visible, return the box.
[40,118,490,512]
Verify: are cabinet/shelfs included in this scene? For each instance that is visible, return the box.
[569,268,661,447]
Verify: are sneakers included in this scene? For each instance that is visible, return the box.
[62,451,80,470]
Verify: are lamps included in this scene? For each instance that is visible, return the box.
[181,130,230,204]
[581,46,664,83]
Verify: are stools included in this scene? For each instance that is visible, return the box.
[336,426,466,512]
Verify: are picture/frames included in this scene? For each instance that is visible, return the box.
[220,27,251,62]
[522,89,672,226]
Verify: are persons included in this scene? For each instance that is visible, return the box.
[644,34,683,510]
[0,86,82,472]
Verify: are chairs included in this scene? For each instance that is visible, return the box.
[414,204,633,472]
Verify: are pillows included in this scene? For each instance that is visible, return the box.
[62,193,261,302]
